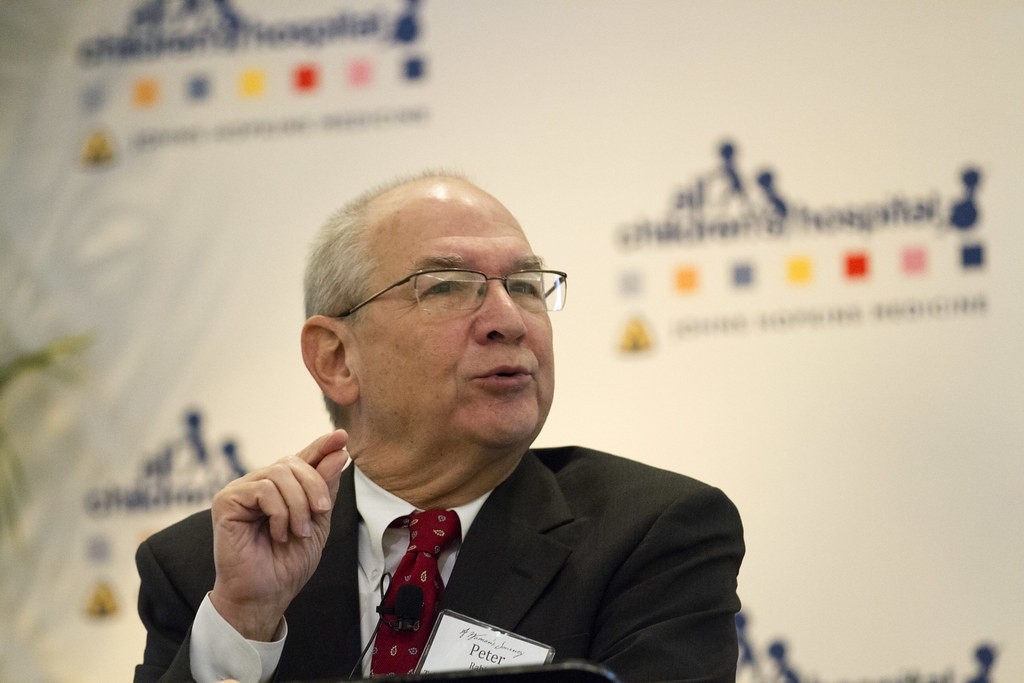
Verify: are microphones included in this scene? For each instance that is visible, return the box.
[391,584,424,631]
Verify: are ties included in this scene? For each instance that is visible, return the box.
[368,509,462,677]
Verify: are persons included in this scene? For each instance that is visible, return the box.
[132,172,746,683]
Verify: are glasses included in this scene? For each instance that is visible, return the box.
[333,268,568,316]
